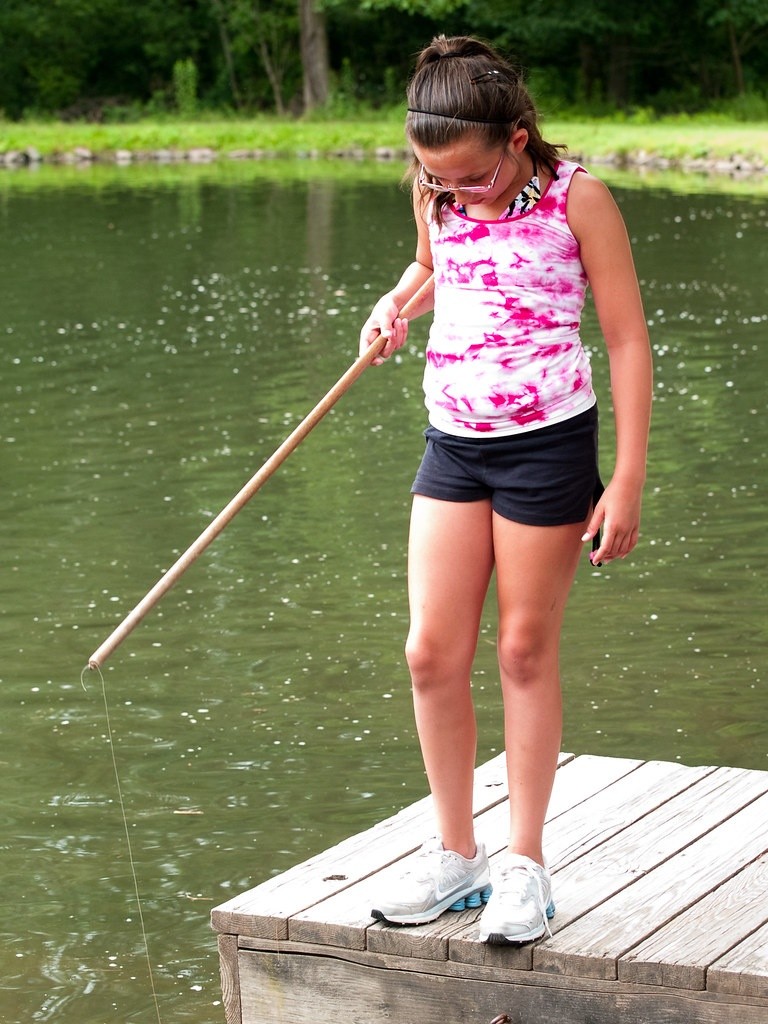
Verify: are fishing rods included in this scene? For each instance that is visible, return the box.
[79,272,435,674]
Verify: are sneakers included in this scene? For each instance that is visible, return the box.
[480,854,556,947]
[371,843,493,927]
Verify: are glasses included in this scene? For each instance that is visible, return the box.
[418,142,508,194]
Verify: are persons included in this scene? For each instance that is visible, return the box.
[360,37,652,946]
